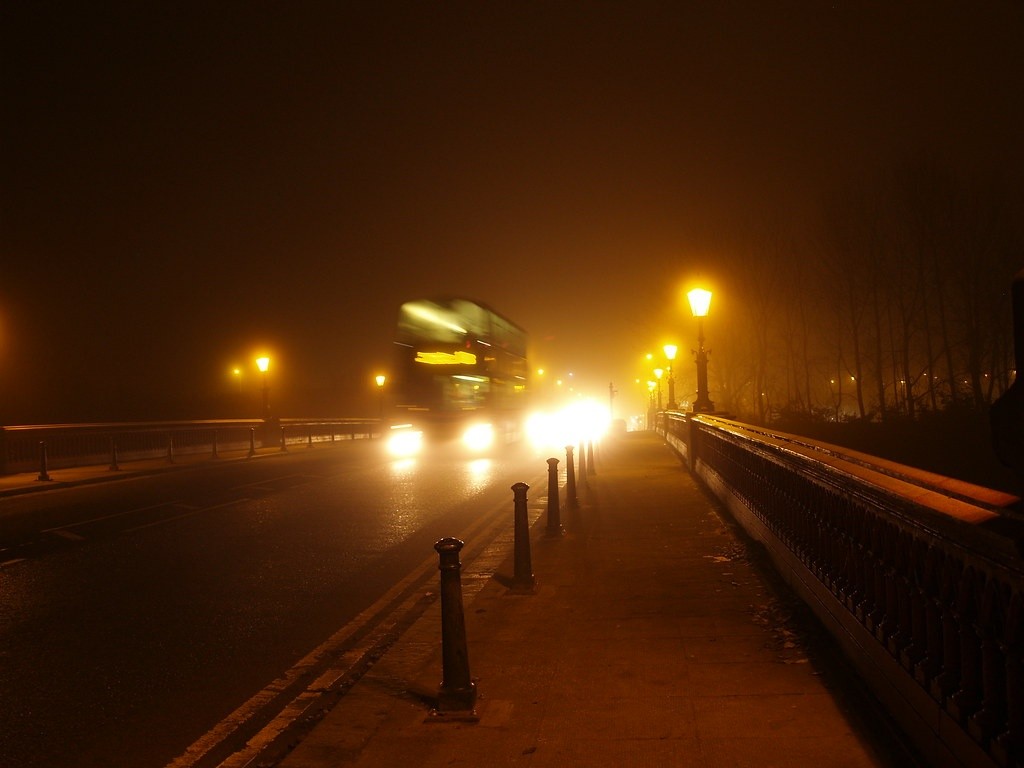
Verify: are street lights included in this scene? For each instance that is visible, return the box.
[663,344,681,412]
[376,374,388,429]
[256,356,281,447]
[645,367,663,413]
[688,288,716,415]
